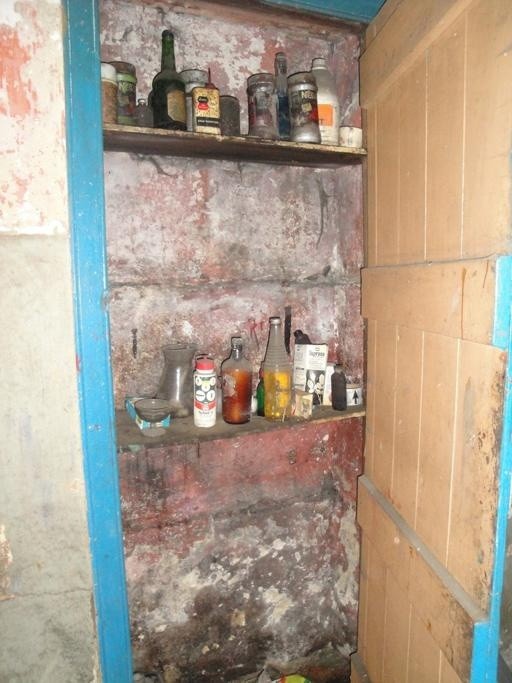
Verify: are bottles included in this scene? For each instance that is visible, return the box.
[132,301,363,439]
[100,28,364,153]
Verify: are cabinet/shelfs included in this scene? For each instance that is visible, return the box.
[105,122,372,453]
